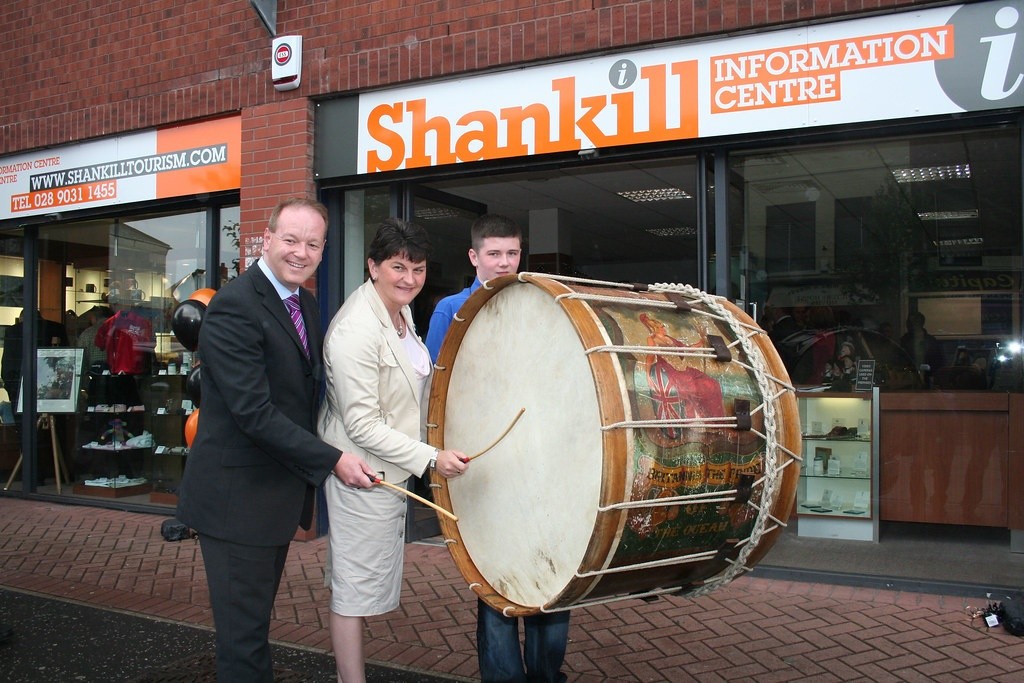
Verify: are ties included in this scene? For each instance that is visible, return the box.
[283,295,311,364]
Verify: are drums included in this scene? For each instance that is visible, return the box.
[773,326,923,392]
[428,272,802,615]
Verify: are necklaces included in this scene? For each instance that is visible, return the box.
[395,312,403,336]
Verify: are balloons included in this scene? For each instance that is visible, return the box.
[189,289,218,309]
[171,300,208,354]
[186,364,204,408]
[183,408,201,448]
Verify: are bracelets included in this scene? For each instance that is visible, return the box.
[430,448,440,471]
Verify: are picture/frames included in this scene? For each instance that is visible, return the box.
[14,346,85,415]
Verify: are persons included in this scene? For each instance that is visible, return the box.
[77,304,146,477]
[0,307,73,487]
[877,310,994,392]
[167,193,381,683]
[317,215,472,683]
[423,215,573,683]
[768,292,796,345]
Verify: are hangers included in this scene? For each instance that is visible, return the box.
[67,302,129,327]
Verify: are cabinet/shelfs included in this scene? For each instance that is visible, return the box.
[70,366,153,500]
[796,386,881,543]
[146,373,201,506]
